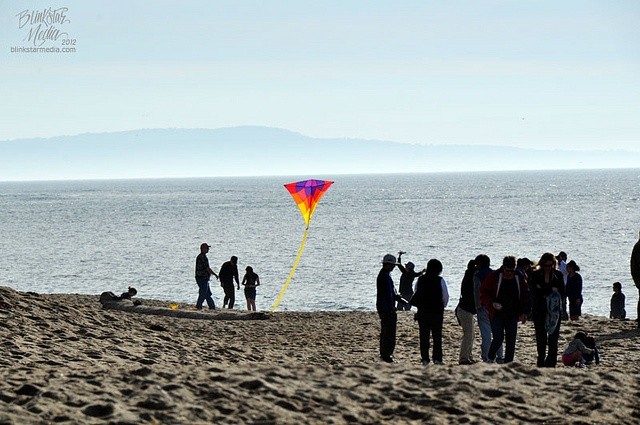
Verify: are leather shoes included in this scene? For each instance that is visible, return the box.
[383,357,399,364]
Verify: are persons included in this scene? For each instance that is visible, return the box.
[566,260,583,320]
[455,259,479,365]
[195,243,218,310]
[474,254,504,364]
[376,254,409,363]
[408,258,449,365]
[609,282,626,320]
[241,266,260,311]
[118,286,137,301]
[555,251,568,320]
[527,253,565,368]
[562,332,600,367]
[396,251,426,311]
[482,256,527,364]
[218,256,240,309]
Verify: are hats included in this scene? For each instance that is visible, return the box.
[405,261,415,272]
[200,243,211,249]
[380,254,400,265]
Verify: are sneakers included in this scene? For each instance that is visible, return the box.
[421,361,429,366]
[459,359,474,366]
[435,359,447,365]
